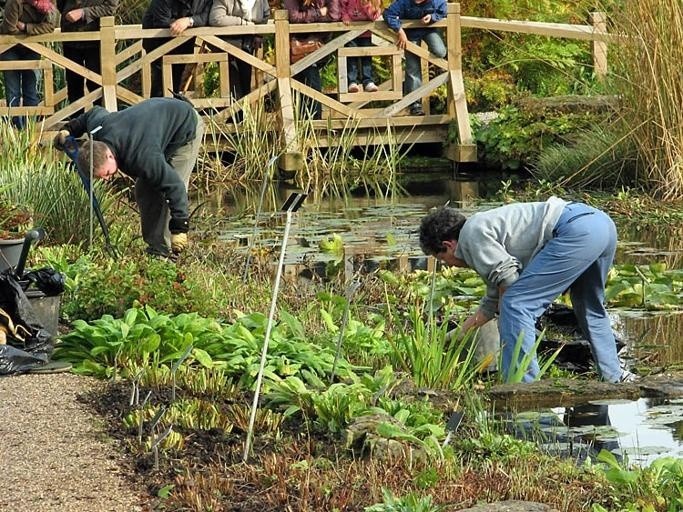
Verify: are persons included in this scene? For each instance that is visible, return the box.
[141,0,212,98]
[282,0,342,118]
[340,0,383,92]
[382,0,447,116]
[419,197,623,386]
[55,0,119,120]
[0,1,56,131]
[208,0,270,123]
[51,96,206,257]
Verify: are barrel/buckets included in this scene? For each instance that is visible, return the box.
[441,312,502,374]
[0,237,26,274]
[24,289,63,343]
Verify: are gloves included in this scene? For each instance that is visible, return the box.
[54,130,69,151]
[170,232,189,257]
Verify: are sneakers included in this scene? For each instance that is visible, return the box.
[365,81,378,91]
[349,82,359,92]
[410,103,425,116]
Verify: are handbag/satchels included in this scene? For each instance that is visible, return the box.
[290,32,325,64]
